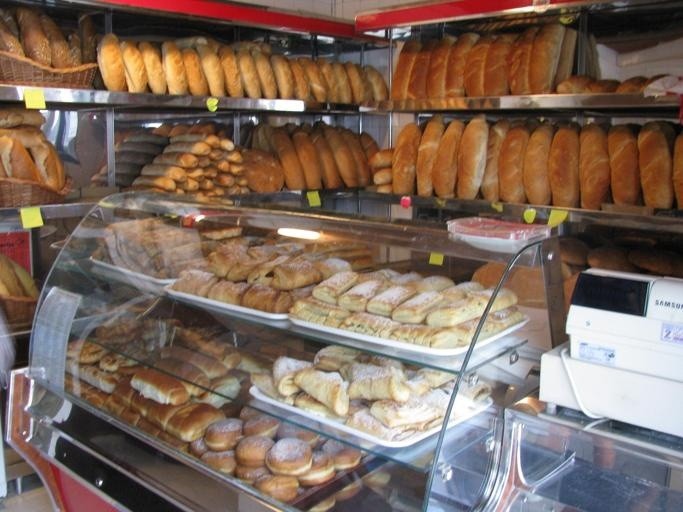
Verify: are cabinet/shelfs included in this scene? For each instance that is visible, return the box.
[0,1,394,497]
[1,188,567,512]
[354,0,682,281]
[481,387,682,512]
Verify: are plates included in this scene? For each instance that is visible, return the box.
[250,386,492,448]
[164,285,290,320]
[289,317,531,355]
[90,256,179,284]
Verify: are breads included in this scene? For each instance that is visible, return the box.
[388,323,467,350]
[90,182,120,188]
[462,34,501,97]
[416,413,444,433]
[272,355,316,396]
[478,119,510,203]
[440,281,484,303]
[346,407,418,442]
[547,121,580,207]
[299,121,313,134]
[286,57,311,100]
[198,179,214,190]
[198,237,251,278]
[373,168,391,185]
[242,410,281,440]
[527,23,565,94]
[234,435,275,467]
[233,465,269,486]
[63,316,180,410]
[487,306,523,332]
[346,376,409,403]
[375,185,392,194]
[321,438,361,470]
[456,114,488,200]
[415,114,445,197]
[287,284,318,301]
[307,494,336,512]
[29,140,74,196]
[292,366,349,417]
[236,48,263,99]
[209,173,234,188]
[368,355,404,374]
[366,285,416,318]
[555,75,596,93]
[174,176,199,192]
[315,59,339,103]
[130,368,188,406]
[368,399,435,428]
[672,134,682,210]
[333,478,362,502]
[0,252,25,298]
[638,73,682,92]
[131,175,176,191]
[92,68,107,90]
[330,59,354,104]
[91,217,210,280]
[523,123,554,206]
[135,418,161,437]
[238,187,249,195]
[167,124,192,137]
[150,123,174,137]
[425,297,493,328]
[281,121,298,136]
[246,254,289,285]
[203,167,218,178]
[637,120,672,210]
[360,131,383,178]
[406,366,457,395]
[321,125,359,188]
[0,2,19,42]
[100,395,125,418]
[203,418,243,451]
[168,133,220,148]
[443,32,480,97]
[169,346,228,380]
[287,295,353,329]
[130,391,153,418]
[199,48,225,97]
[77,13,97,66]
[466,287,518,314]
[214,186,224,197]
[297,451,335,486]
[311,271,358,306]
[220,183,240,196]
[228,40,257,50]
[152,152,199,168]
[209,159,230,174]
[577,122,611,211]
[0,15,25,58]
[342,60,365,106]
[249,371,297,406]
[239,401,272,424]
[170,268,219,298]
[362,471,392,488]
[202,190,217,197]
[122,133,169,145]
[136,187,185,196]
[425,35,458,97]
[114,151,156,164]
[10,257,40,301]
[234,176,248,187]
[339,128,372,188]
[180,333,242,371]
[161,40,188,96]
[337,278,386,313]
[183,190,205,198]
[353,62,375,108]
[295,391,359,425]
[67,30,82,68]
[312,121,328,133]
[552,26,576,93]
[440,379,492,404]
[301,240,353,255]
[453,316,495,341]
[180,46,208,97]
[98,163,139,176]
[361,64,388,103]
[268,261,321,291]
[406,35,438,99]
[0,107,46,129]
[111,376,137,408]
[162,141,210,155]
[225,246,270,282]
[223,150,242,163]
[338,361,404,383]
[166,403,226,442]
[151,357,208,397]
[483,32,516,96]
[120,407,141,427]
[219,138,234,151]
[254,39,271,52]
[38,13,71,69]
[507,25,541,95]
[614,75,648,93]
[382,273,422,288]
[251,122,274,152]
[208,149,227,161]
[366,148,396,168]
[113,141,164,156]
[218,119,257,148]
[249,46,278,99]
[312,247,374,272]
[390,37,428,100]
[218,45,244,98]
[498,126,530,204]
[146,400,198,432]
[264,437,312,475]
[137,41,167,96]
[140,163,187,183]
[403,276,455,295]
[187,436,208,459]
[275,414,320,448]
[391,122,422,194]
[206,278,252,305]
[215,122,229,138]
[119,40,148,93]
[607,123,641,207]
[268,53,293,99]
[431,119,463,198]
[580,78,620,92]
[229,163,245,177]
[190,374,241,410]
[184,118,218,134]
[0,125,46,149]
[90,174,130,187]
[199,449,235,475]
[290,128,322,189]
[234,144,284,192]
[183,167,204,183]
[240,283,291,314]
[253,473,299,502]
[337,311,401,338]
[15,6,51,68]
[268,128,307,191]
[310,258,352,282]
[195,155,211,168]
[334,124,346,132]
[157,431,189,453]
[308,128,345,188]
[233,348,275,375]
[391,291,443,324]
[96,32,125,91]
[297,55,327,103]
[0,134,44,184]
